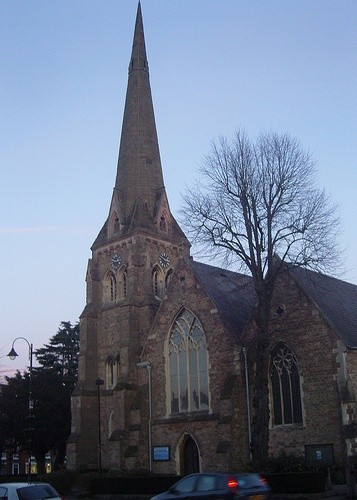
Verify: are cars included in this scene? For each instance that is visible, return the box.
[1,455,51,474]
[0,482,61,500]
[150,471,271,500]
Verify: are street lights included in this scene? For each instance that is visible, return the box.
[7,337,33,475]
[95,378,104,472]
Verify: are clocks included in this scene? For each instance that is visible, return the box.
[112,253,121,269]
[159,253,171,268]
